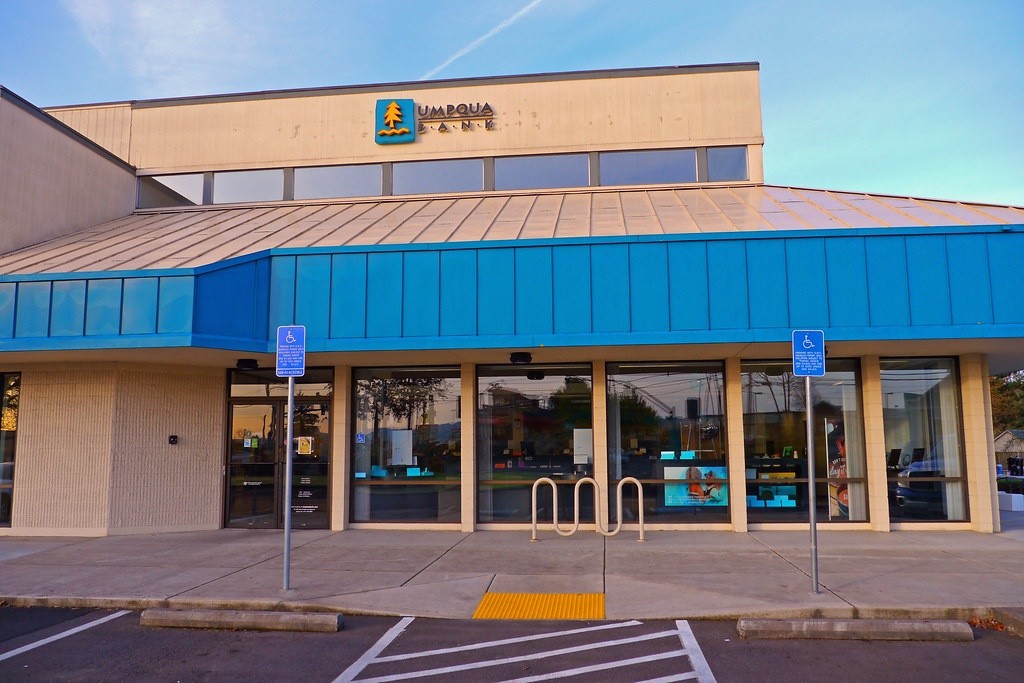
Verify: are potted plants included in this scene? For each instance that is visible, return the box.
[997,477,1024,512]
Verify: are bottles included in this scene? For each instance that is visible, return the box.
[772,454,780,466]
[243,428,258,449]
[762,454,770,467]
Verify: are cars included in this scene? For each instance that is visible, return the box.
[0,462,15,521]
[897,441,947,515]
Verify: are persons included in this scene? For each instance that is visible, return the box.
[830,425,848,518]
[686,466,719,502]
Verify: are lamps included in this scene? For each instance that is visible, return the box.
[235,359,259,369]
[509,352,532,364]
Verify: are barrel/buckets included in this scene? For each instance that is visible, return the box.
[1002,470,1009,476]
[997,465,1002,474]
